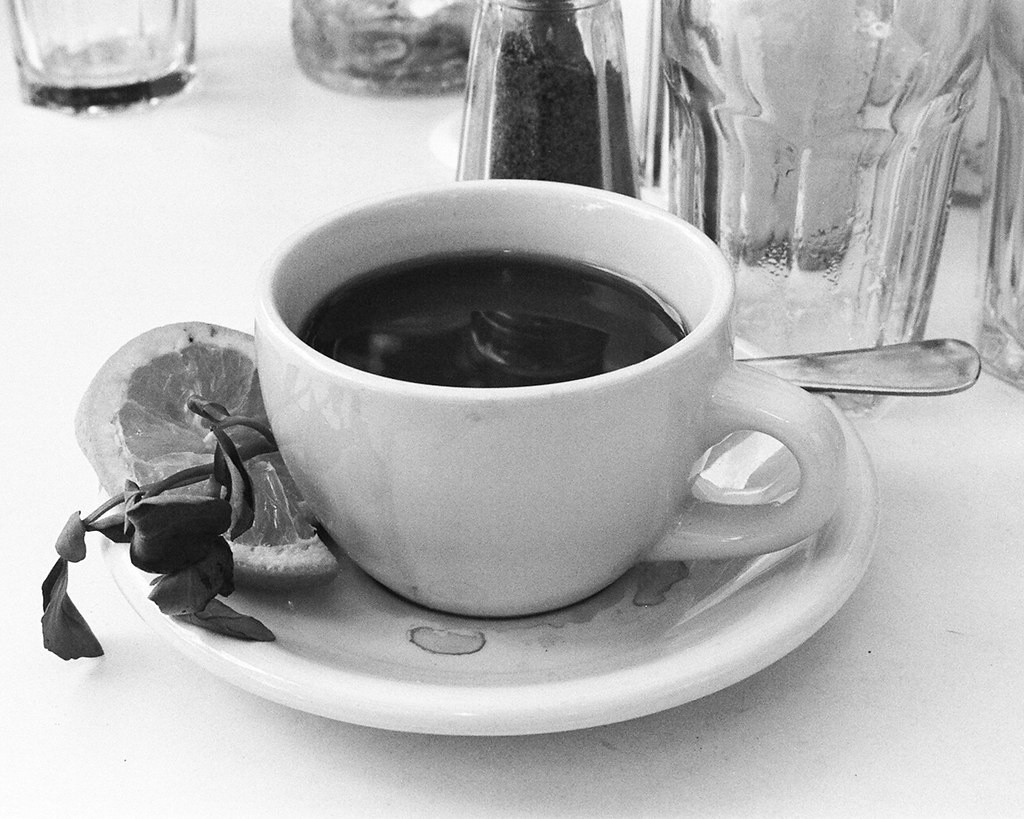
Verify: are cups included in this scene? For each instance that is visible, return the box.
[456,0,642,202]
[293,0,480,97]
[979,0,1024,391]
[6,0,196,116]
[661,0,989,415]
[254,178,846,618]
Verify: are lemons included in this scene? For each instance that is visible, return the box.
[75,322,340,588]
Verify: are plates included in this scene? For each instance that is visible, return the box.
[105,339,878,736]
